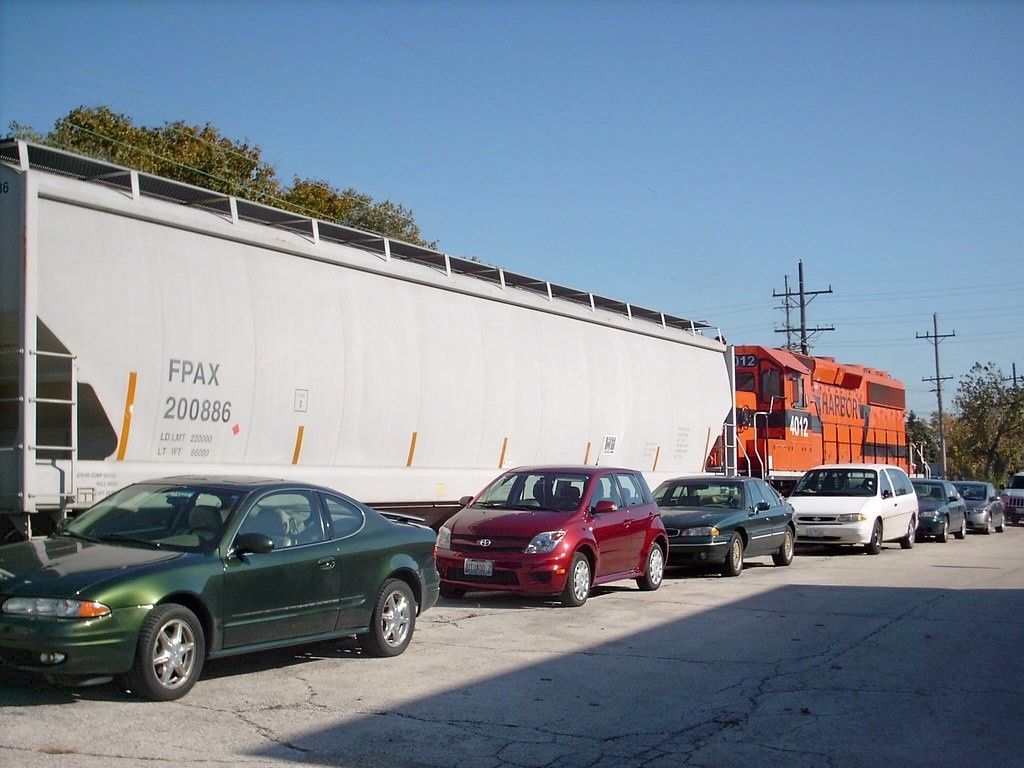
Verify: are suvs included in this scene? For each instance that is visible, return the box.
[999,471,1024,525]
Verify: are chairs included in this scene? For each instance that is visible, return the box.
[583,478,604,509]
[560,486,580,501]
[930,487,942,497]
[678,496,700,506]
[976,491,986,500]
[174,505,224,541]
[862,478,876,492]
[255,507,299,547]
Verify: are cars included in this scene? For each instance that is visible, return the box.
[908,478,968,543]
[949,480,1005,535]
[0,474,442,701]
[645,474,796,577]
[434,462,670,607]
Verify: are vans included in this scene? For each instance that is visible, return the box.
[786,463,921,556]
